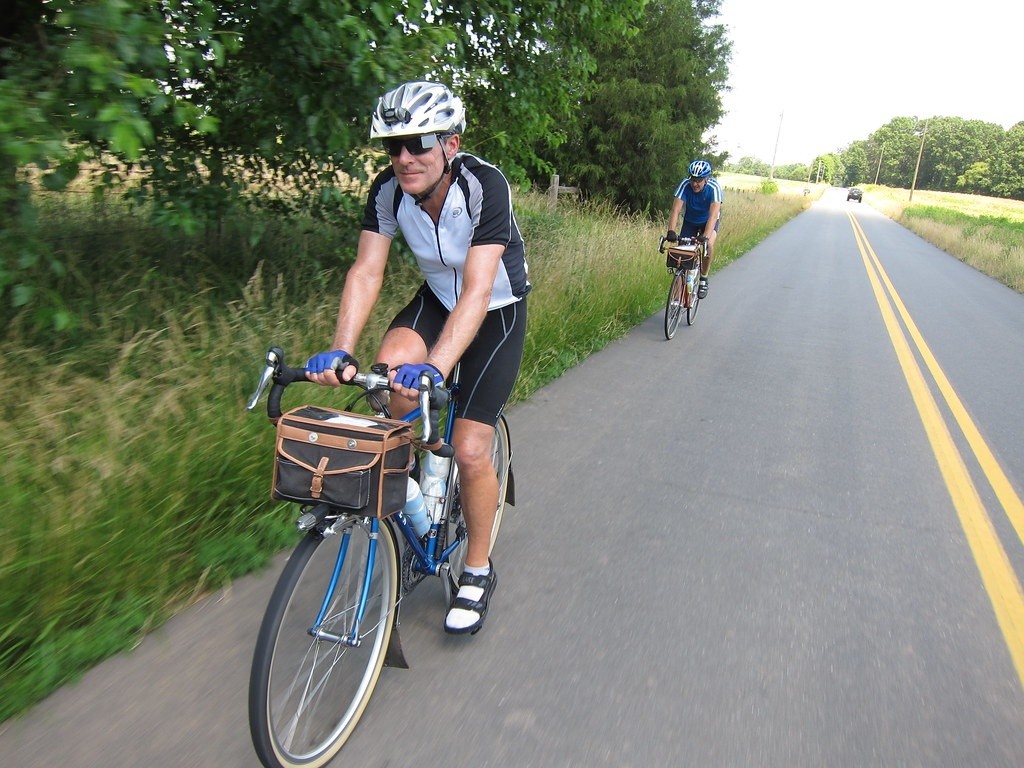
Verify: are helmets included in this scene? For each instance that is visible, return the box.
[687,160,712,178]
[367,79,468,138]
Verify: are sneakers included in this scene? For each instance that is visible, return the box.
[697,276,709,299]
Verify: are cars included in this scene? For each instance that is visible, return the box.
[847,187,864,204]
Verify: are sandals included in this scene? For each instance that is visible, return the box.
[443,555,499,635]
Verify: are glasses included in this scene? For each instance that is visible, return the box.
[690,176,705,183]
[378,131,454,156]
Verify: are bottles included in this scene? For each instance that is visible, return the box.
[686,275,693,293]
[419,437,451,525]
[688,267,698,280]
[403,475,431,538]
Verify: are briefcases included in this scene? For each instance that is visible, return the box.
[269,403,415,520]
[665,244,698,270]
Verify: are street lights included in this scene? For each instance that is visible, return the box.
[769,110,785,188]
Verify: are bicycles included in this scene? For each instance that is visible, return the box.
[238,344,515,768]
[651,231,710,343]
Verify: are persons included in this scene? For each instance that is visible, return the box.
[666,161,724,318]
[303,81,534,634]
